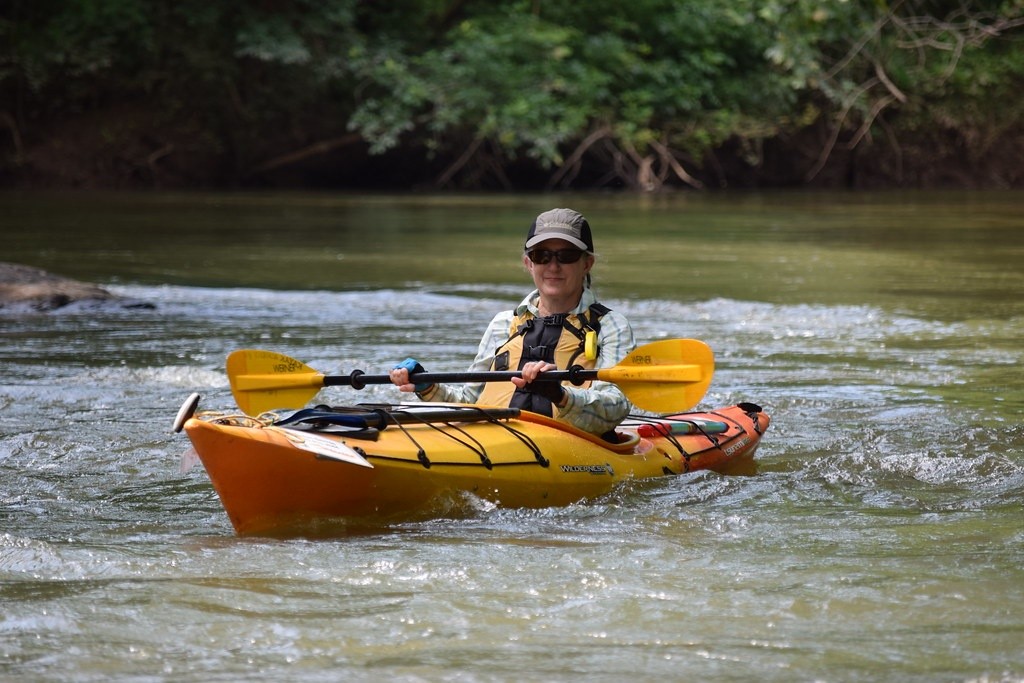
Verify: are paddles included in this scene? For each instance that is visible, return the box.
[224,334,717,416]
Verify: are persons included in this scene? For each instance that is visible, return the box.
[390,208,638,437]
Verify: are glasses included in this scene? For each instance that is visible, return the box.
[526,249,583,264]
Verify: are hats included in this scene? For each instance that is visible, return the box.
[524,208,594,254]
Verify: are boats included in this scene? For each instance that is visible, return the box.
[170,392,770,538]
